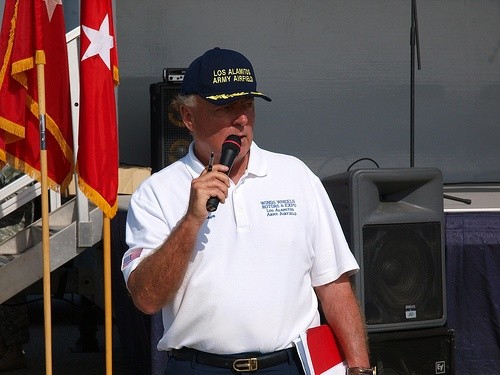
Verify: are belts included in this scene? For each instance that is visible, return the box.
[169,348,299,373]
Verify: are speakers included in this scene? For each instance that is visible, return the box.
[319,168,456,375]
[150,81,195,177]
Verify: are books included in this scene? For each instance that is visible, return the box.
[293,323,350,375]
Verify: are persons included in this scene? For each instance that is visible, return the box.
[120,47,376,375]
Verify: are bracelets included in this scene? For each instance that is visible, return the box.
[347,366,377,375]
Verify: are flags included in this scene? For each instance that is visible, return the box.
[75,0,119,219]
[0,0,75,193]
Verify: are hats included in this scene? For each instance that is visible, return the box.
[179,47,272,103]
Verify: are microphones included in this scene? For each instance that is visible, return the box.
[207,135,242,212]
